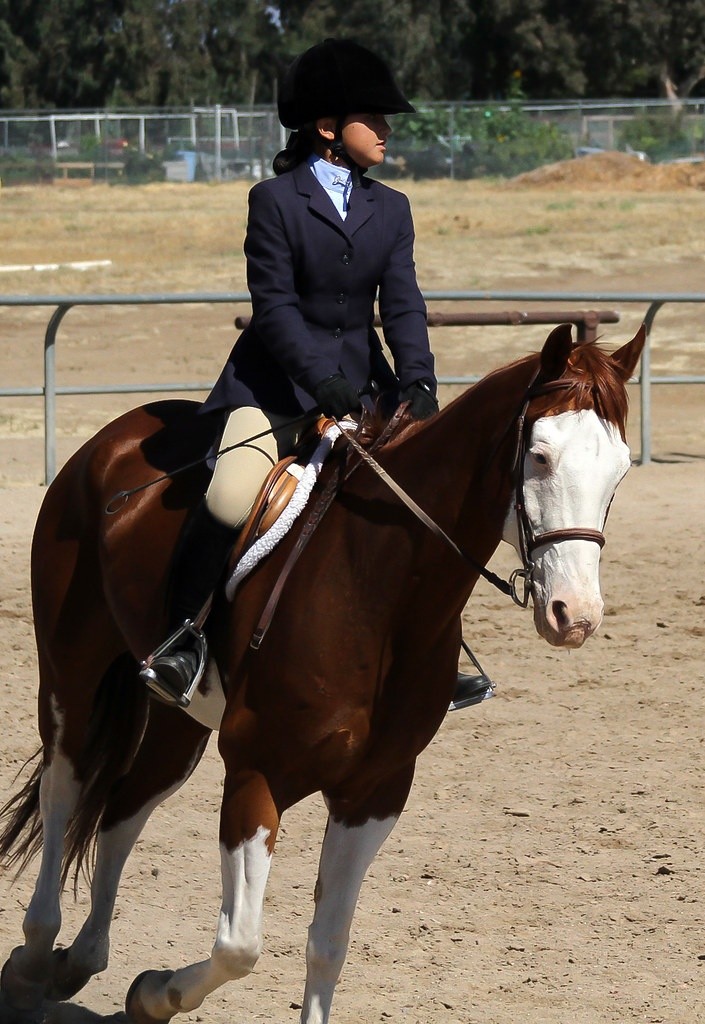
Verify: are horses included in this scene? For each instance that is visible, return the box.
[0,324,646,1024]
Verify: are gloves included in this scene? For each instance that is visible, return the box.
[312,374,362,420]
[401,381,439,421]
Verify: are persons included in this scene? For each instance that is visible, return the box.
[151,37,488,709]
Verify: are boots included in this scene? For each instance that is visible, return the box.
[152,495,244,695]
[453,670,490,701]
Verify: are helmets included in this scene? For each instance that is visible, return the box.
[278,39,416,128]
